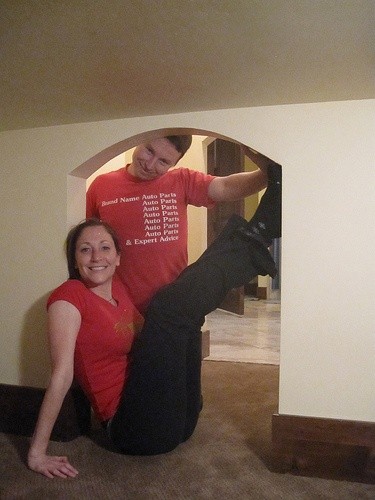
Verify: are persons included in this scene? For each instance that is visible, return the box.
[83,133,273,319]
[25,165,284,481]
